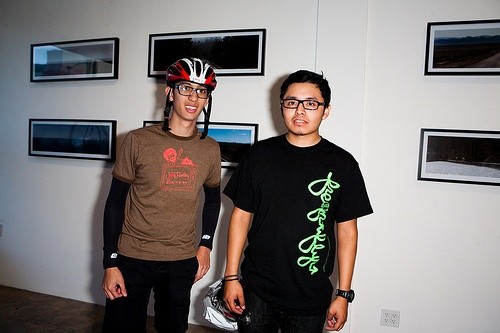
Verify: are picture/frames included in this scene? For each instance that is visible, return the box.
[148,27,266,77]
[417,127,500,186]
[424,19,500,77]
[143,121,260,167]
[29,119,117,162]
[28,37,120,83]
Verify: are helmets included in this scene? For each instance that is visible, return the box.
[166,57,217,91]
[201,276,252,332]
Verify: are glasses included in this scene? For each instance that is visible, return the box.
[280,98,326,110]
[169,82,211,99]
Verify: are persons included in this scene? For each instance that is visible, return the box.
[224,69,373,333]
[103,57,222,333]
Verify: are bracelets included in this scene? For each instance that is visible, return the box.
[224,277,239,282]
[224,274,238,278]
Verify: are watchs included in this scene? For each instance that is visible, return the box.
[336,289,355,303]
